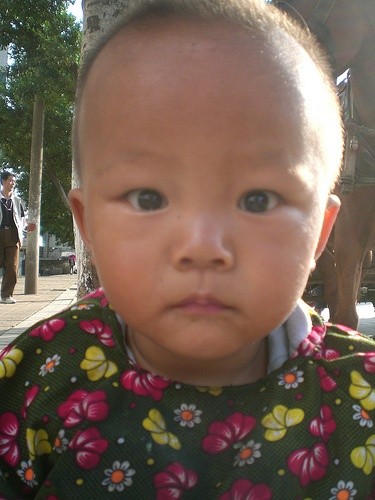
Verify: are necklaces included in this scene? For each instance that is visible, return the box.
[0,199,12,210]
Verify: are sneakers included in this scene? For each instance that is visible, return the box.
[0,297,16,304]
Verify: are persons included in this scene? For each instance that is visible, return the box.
[0,0,375,500]
[68,257,76,275]
[268,0,375,331]
[0,171,26,304]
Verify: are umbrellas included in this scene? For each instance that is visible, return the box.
[68,255,76,259]
